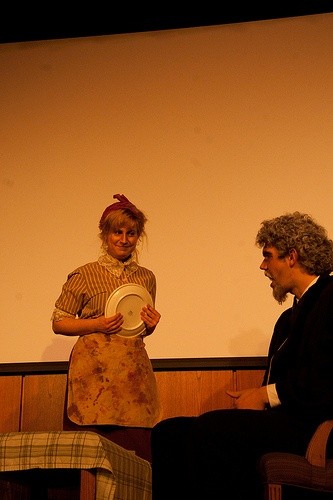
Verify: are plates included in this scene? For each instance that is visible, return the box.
[105,283,153,338]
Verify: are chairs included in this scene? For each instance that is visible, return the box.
[249,416,333,500]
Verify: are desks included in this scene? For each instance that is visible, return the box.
[1,431,153,500]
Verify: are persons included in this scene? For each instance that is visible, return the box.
[149,211,333,500]
[51,195,163,462]
